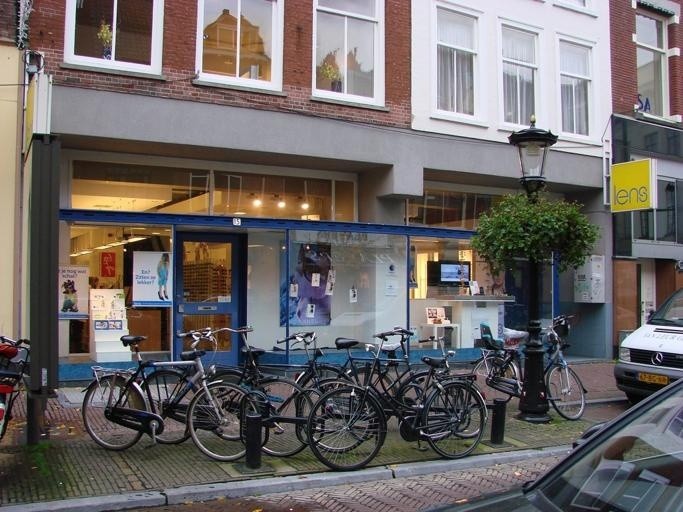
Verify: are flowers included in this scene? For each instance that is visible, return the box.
[319,59,341,81]
[97,18,113,49]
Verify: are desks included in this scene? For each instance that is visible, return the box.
[419,323,461,349]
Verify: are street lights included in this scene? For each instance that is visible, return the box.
[506,115,559,412]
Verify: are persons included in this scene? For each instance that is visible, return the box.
[157,253,170,300]
[62,283,79,312]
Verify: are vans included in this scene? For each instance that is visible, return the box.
[614,289,682,404]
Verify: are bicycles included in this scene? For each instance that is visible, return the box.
[0,336,30,440]
[469,314,586,420]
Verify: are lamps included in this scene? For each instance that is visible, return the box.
[70,228,147,256]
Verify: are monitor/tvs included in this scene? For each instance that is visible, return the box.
[426,261,439,288]
[439,260,472,287]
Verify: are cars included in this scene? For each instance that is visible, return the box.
[423,380,683,511]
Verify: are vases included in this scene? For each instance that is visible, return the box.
[331,80,341,92]
[101,47,112,60]
